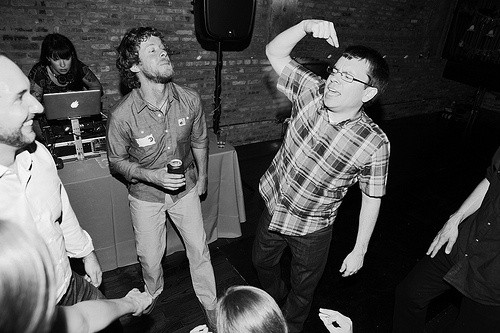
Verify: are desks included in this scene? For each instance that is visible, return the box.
[54,127,246,273]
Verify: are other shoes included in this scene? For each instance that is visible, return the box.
[142,295,160,315]
[196,296,219,330]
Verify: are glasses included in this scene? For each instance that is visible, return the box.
[327,65,374,87]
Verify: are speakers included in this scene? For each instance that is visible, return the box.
[193,0,256,52]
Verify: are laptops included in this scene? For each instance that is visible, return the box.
[43,87,101,119]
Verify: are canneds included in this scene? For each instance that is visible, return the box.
[167,159,182,175]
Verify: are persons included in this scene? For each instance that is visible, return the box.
[107,27,220,333]
[26,33,109,157]
[0,51,354,333]
[250,20,391,333]
[412,151,500,333]
